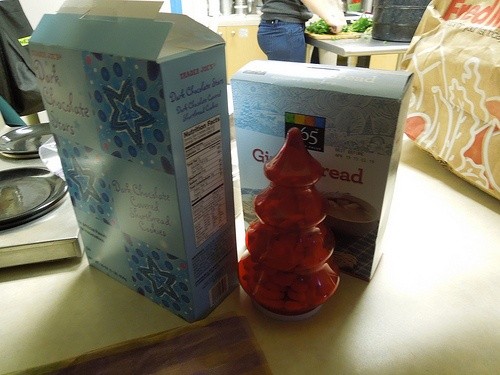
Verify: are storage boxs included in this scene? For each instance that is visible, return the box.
[231,61,415,282]
[29,1,239,323]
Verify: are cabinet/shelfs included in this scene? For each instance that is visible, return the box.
[217,25,268,84]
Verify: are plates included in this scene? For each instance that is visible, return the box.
[0,167,69,225]
[0,123,52,154]
[252,299,322,323]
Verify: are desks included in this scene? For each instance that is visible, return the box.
[305,35,410,66]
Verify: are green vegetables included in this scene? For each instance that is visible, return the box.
[306,18,331,33]
[343,16,373,32]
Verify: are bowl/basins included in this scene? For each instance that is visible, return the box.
[323,192,379,236]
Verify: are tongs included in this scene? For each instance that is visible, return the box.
[304,29,360,40]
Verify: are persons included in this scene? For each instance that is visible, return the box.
[257,0,341,63]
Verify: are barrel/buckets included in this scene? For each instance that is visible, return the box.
[372,0,432,41]
